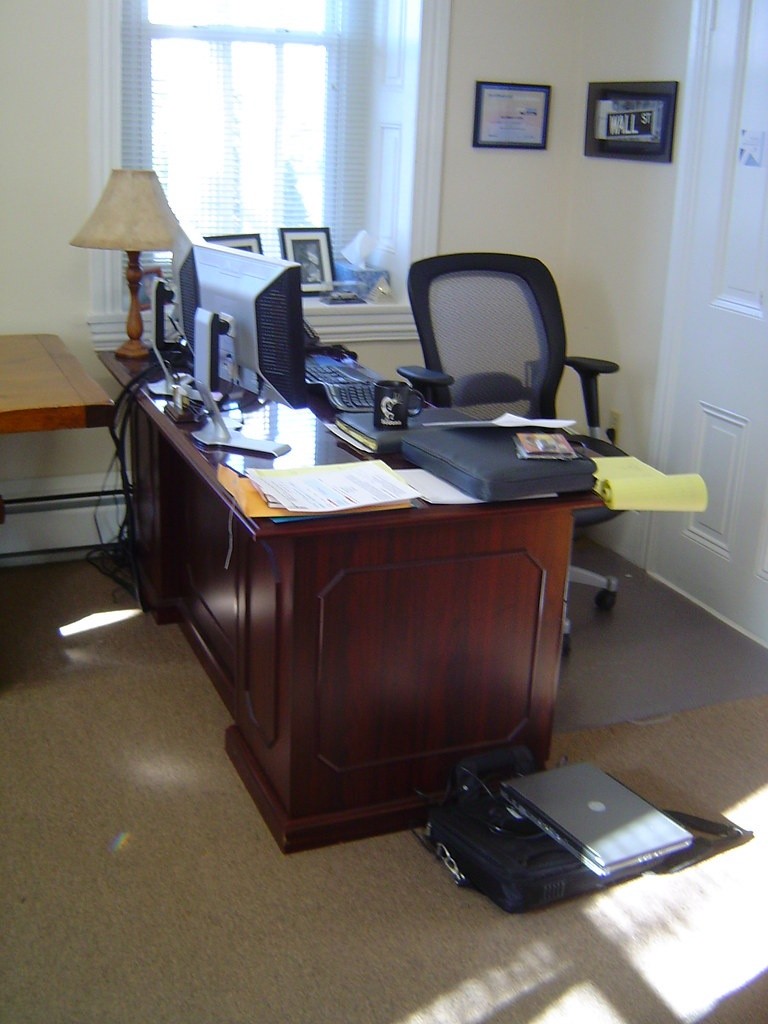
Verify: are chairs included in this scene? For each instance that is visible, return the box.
[396,252,630,653]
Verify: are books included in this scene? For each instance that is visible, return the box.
[334,405,480,455]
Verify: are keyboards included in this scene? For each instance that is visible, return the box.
[305,353,379,412]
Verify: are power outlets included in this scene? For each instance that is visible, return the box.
[609,410,621,444]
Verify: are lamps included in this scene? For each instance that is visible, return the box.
[70,170,189,359]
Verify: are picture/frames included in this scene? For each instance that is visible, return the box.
[583,80,677,164]
[473,80,551,150]
[203,234,263,256]
[280,226,336,298]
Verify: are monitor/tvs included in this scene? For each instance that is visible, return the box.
[171,236,307,457]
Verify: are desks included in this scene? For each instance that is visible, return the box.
[95,351,609,853]
[0,332,152,618]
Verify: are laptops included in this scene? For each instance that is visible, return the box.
[499,760,693,876]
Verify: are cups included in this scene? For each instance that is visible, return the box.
[373,381,424,430]
